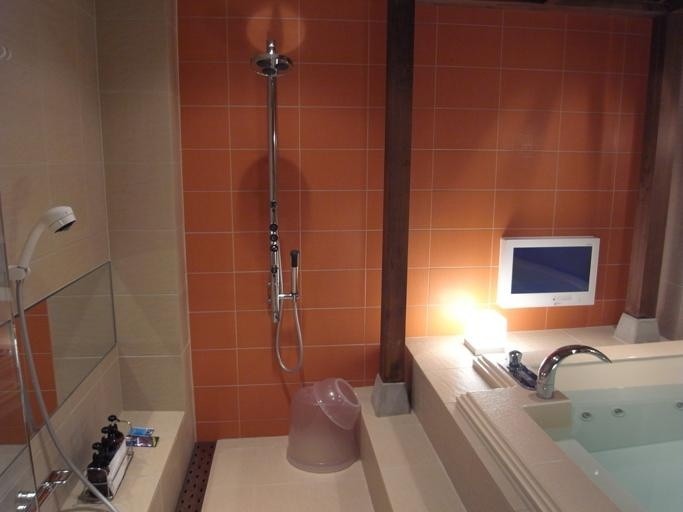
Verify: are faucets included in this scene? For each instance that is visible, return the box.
[534,343,611,399]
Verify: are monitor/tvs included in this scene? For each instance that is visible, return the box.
[497,236,600,310]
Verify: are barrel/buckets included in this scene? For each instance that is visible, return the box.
[286,389,360,474]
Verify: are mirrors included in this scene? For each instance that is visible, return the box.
[0,261,113,479]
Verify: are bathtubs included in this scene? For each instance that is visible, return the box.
[457,338,683,511]
